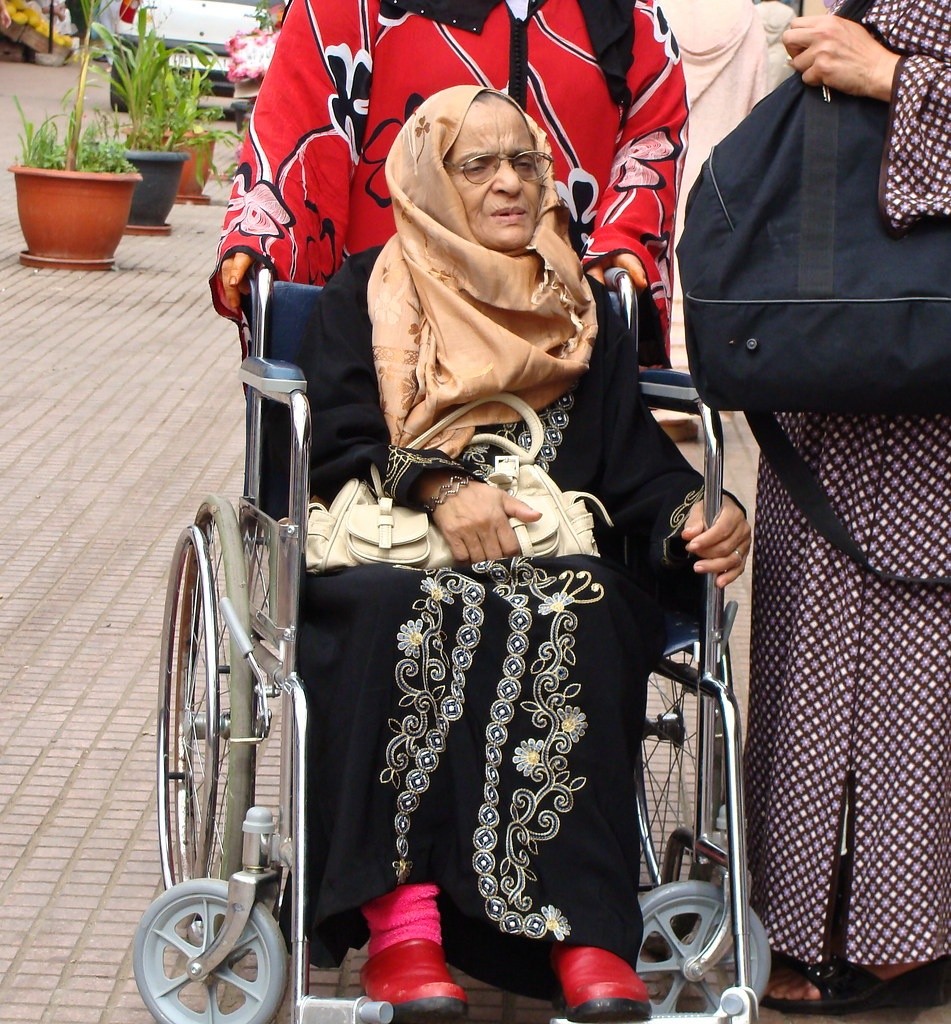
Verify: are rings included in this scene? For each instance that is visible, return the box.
[733,550,742,562]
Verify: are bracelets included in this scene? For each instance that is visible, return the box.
[425,474,474,511]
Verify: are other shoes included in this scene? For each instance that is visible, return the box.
[551,940,652,1023]
[359,939,468,1024]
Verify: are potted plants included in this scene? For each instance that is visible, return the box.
[120,64,218,206]
[5,56,143,271]
[86,6,245,238]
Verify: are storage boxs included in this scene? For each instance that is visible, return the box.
[0,42,26,62]
[0,20,26,42]
[19,25,75,60]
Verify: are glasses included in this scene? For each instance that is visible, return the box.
[442,151,553,185]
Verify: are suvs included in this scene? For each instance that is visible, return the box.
[103,1,271,117]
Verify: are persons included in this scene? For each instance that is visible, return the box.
[0,0,76,65]
[263,81,753,1024]
[208,0,689,402]
[649,0,803,441]
[746,1,951,1015]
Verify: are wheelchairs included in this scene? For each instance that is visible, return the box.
[131,260,777,1024]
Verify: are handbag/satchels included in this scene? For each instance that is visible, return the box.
[674,0,951,412]
[302,392,613,574]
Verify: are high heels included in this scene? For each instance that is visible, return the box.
[759,953,951,1015]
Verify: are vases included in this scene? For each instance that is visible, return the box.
[230,101,254,134]
